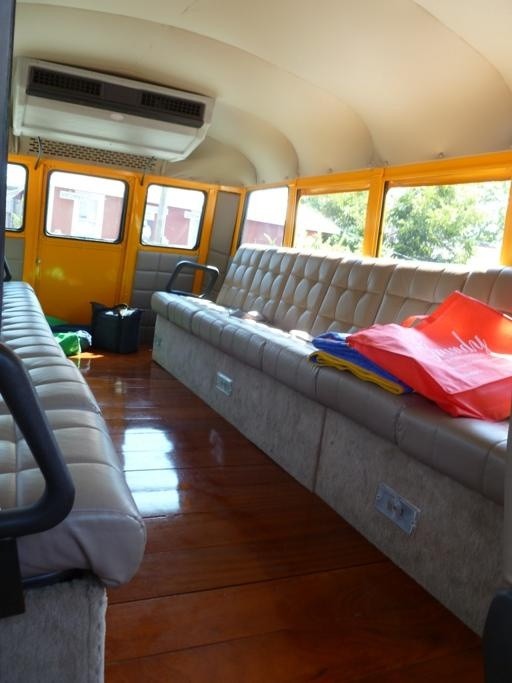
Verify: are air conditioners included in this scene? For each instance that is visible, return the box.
[9,55,218,163]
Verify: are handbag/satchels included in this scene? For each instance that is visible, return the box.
[347,291,512,421]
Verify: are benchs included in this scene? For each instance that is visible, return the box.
[148,243,511,639]
[0,244,146,681]
